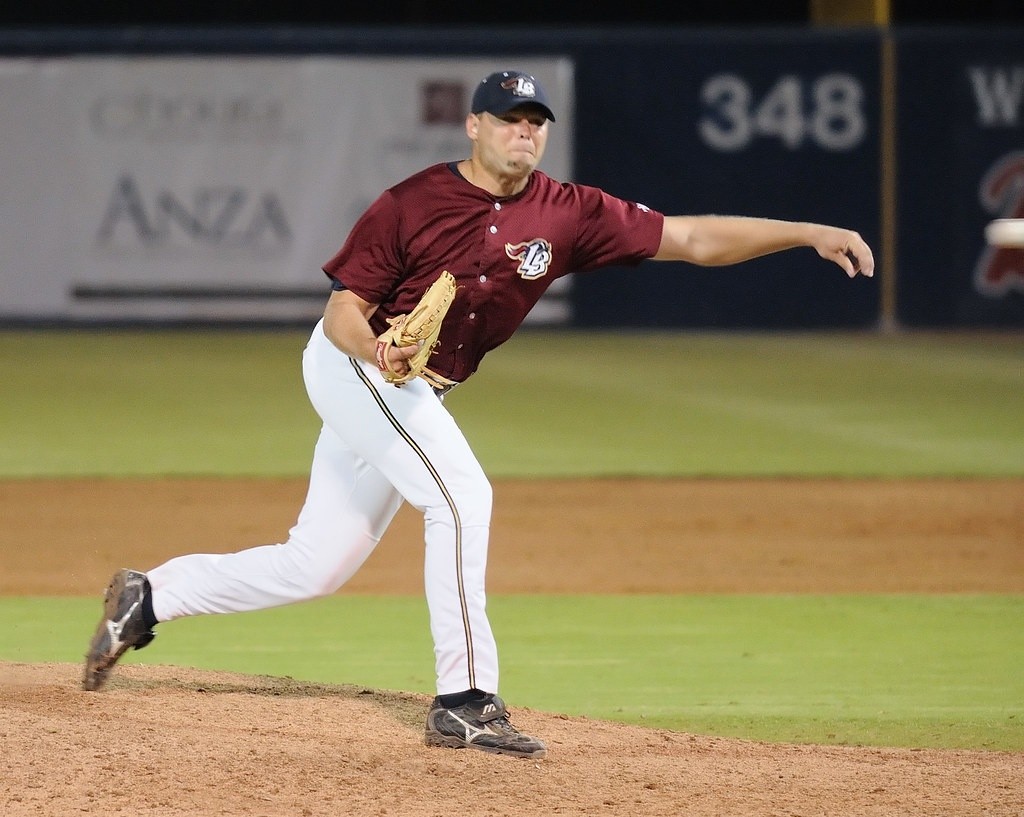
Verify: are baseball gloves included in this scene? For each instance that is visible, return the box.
[375,269,458,388]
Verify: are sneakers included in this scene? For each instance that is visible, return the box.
[83,567,157,690]
[424,691,546,759]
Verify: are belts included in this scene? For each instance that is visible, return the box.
[425,374,456,397]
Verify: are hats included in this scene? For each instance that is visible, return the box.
[471,71,556,123]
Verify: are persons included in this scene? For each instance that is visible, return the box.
[82,68,876,758]
